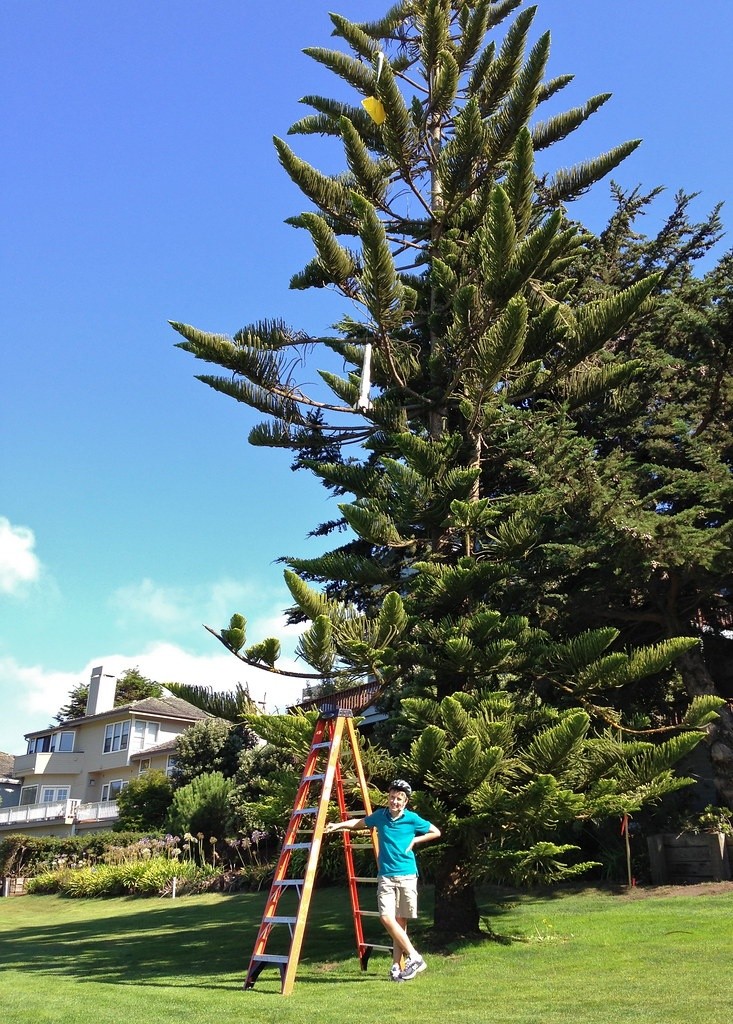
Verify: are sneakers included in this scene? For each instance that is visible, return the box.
[402,955,427,979]
[390,963,405,982]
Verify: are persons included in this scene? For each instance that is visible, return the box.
[322,779,441,981]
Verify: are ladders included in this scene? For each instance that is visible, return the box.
[244,707,404,997]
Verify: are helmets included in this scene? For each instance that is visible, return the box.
[389,779,412,798]
